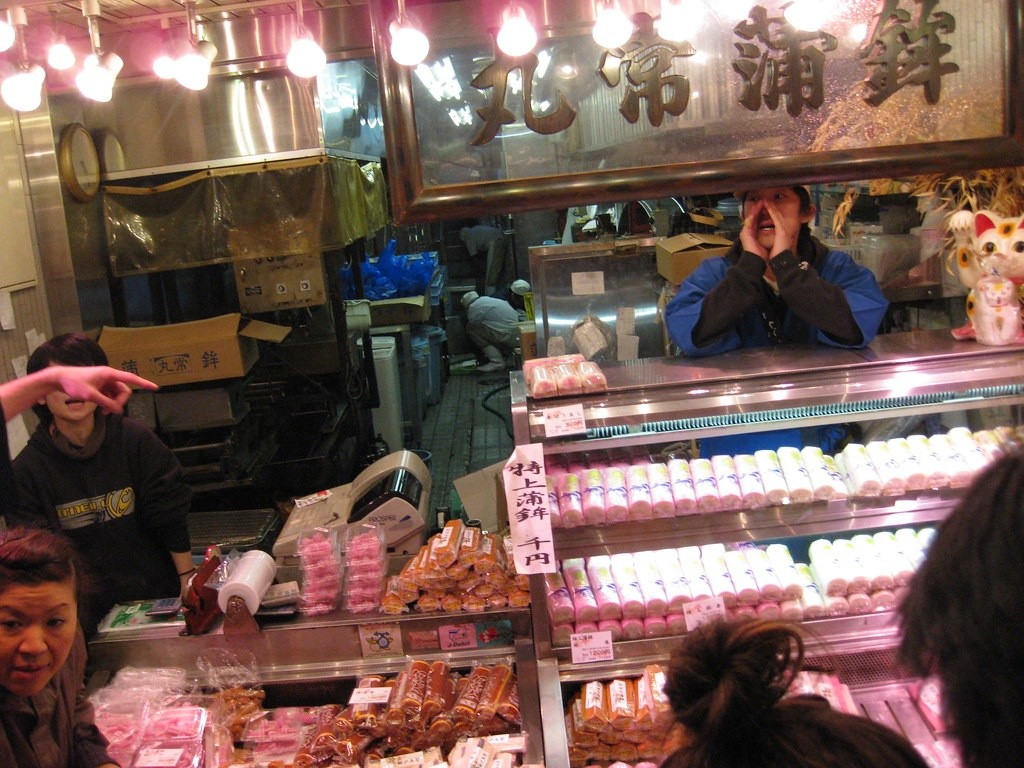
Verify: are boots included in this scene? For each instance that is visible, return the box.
[476,345,506,372]
[486,287,496,296]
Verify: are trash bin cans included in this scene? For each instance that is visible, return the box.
[411,325,447,406]
[410,335,430,421]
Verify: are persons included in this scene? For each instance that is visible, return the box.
[461,225,507,294]
[890,447,1024,768]
[0,333,199,648]
[510,281,531,323]
[616,195,719,235]
[0,529,122,768]
[659,616,927,768]
[460,290,520,371]
[662,183,890,567]
[0,365,159,426]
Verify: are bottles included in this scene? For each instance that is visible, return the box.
[466,519,481,534]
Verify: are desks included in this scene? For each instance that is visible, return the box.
[370,325,421,447]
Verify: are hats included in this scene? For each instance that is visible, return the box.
[460,291,479,309]
[733,184,811,201]
[458,227,471,241]
[510,279,530,296]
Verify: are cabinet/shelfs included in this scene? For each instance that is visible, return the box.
[510,329,1024,768]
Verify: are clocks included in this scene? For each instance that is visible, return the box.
[60,123,100,202]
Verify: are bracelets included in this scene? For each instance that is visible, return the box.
[179,568,196,576]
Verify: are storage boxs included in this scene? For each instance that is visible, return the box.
[517,324,537,363]
[655,233,735,285]
[346,300,371,336]
[369,269,441,326]
[98,314,293,390]
[820,233,921,287]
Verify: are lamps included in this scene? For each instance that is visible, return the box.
[390,0,430,66]
[150,0,218,90]
[497,0,537,57]
[2,6,75,112]
[72,0,123,103]
[287,0,326,78]
[591,0,632,48]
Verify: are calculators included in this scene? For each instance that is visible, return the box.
[145,597,183,616]
